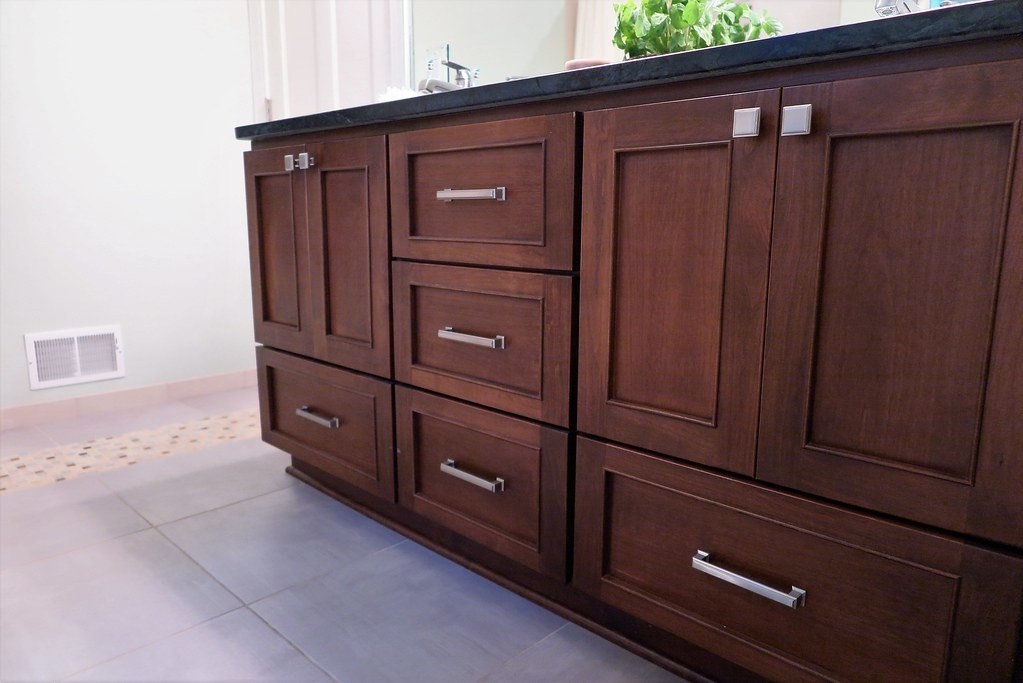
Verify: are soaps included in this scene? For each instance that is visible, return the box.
[564,57,612,70]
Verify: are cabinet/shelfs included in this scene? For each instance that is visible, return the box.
[245,30,1023,683]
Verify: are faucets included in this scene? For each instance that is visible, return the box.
[417,57,474,95]
